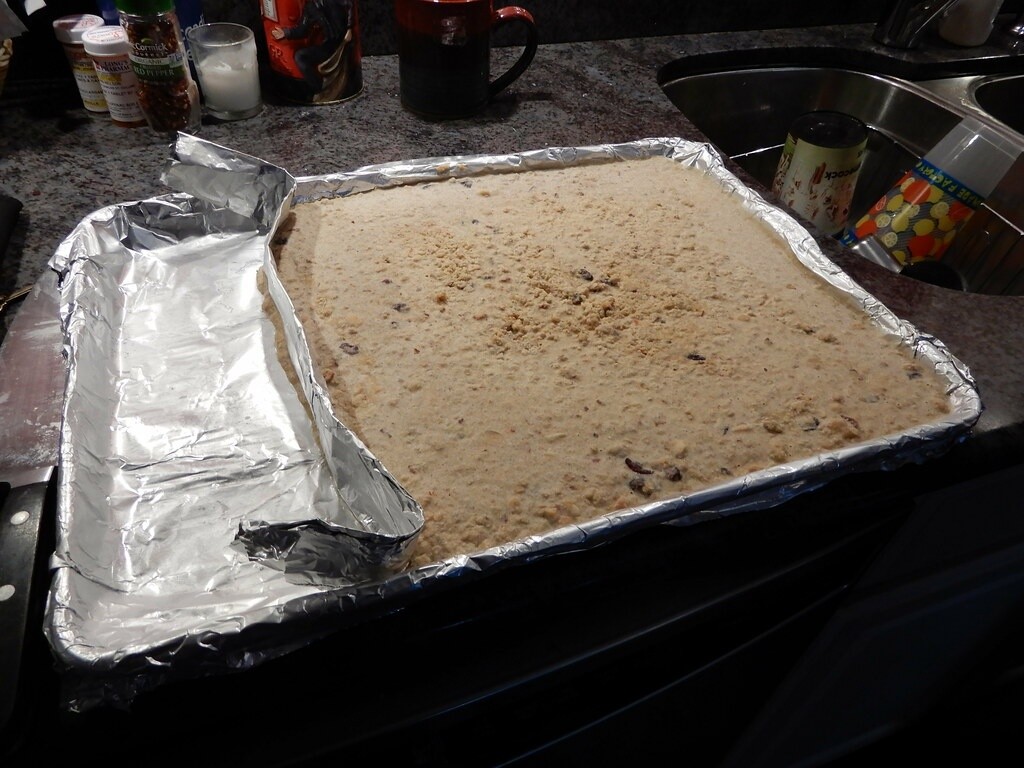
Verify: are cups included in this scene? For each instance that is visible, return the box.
[186,22,264,123]
[260,1,365,106]
[771,110,870,242]
[939,0,1004,47]
[395,0,539,120]
[836,111,1024,276]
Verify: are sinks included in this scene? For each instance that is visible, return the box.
[655,67,1024,319]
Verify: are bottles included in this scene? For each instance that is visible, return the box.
[82,25,148,130]
[53,15,110,114]
[114,1,204,140]
